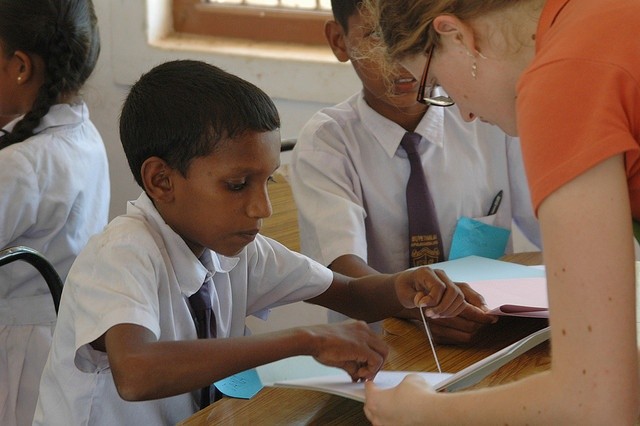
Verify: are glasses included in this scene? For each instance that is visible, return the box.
[415,45,454,108]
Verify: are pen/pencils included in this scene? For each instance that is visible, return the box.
[487,190,503,215]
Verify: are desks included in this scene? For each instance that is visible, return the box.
[174,252,549,425]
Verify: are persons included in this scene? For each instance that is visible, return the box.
[288,0,544,348]
[1,1,112,426]
[32,59,468,426]
[362,0,640,426]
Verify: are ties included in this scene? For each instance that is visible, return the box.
[400,133,444,268]
[191,280,221,407]
